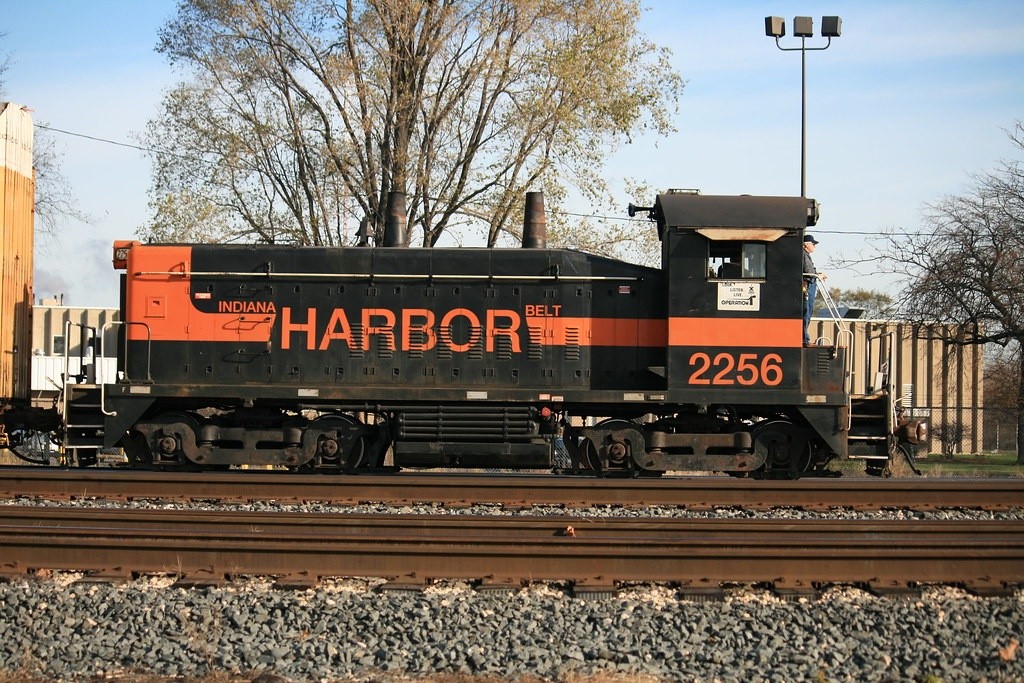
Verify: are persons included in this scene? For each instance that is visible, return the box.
[803,235,827,346]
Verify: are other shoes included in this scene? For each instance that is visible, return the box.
[803,343,809,348]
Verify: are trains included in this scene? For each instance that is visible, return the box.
[53,188,927,480]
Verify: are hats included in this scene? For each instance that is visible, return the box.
[804,235,819,244]
